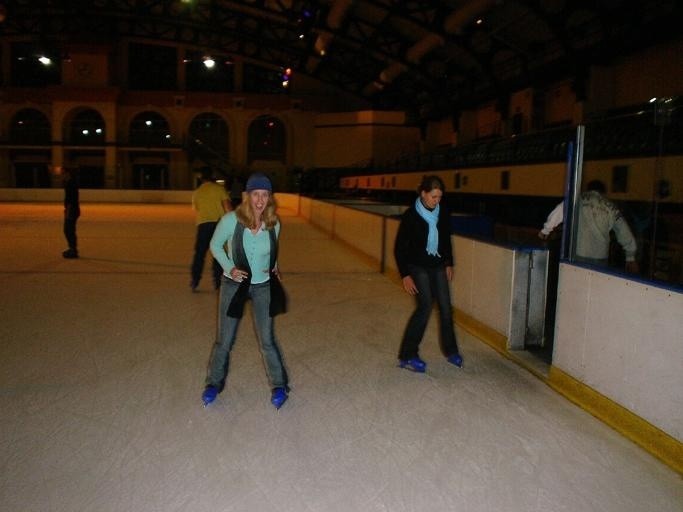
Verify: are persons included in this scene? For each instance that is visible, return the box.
[538,179,638,274]
[191,167,233,290]
[58,165,81,257]
[393,175,462,372]
[200,172,290,407]
[222,169,247,209]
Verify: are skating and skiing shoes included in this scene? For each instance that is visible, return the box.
[447,353,462,368]
[397,356,427,373]
[270,387,288,411]
[190,279,200,291]
[201,386,218,407]
[62,249,78,258]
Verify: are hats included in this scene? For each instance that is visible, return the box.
[245,170,272,191]
